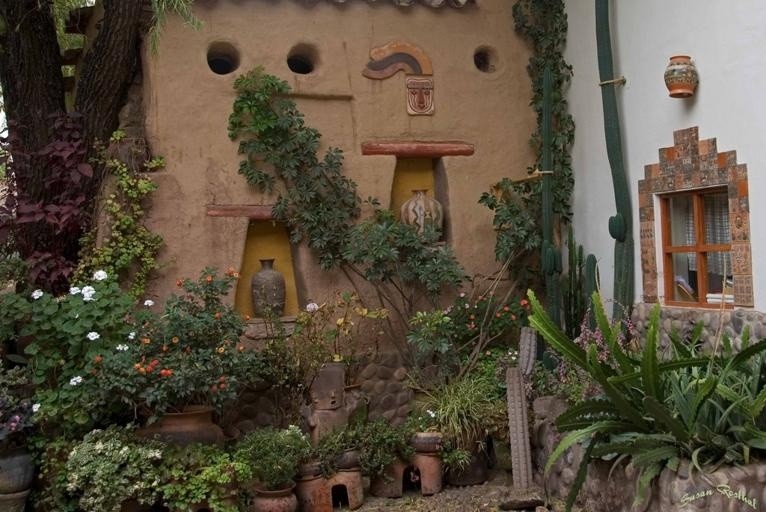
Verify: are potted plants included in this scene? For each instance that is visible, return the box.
[232,425,374,511]
[413,379,500,487]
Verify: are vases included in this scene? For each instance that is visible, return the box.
[1,449,42,493]
[663,56,698,99]
[1,269,265,446]
[147,404,226,452]
[249,256,286,318]
[399,186,443,242]
